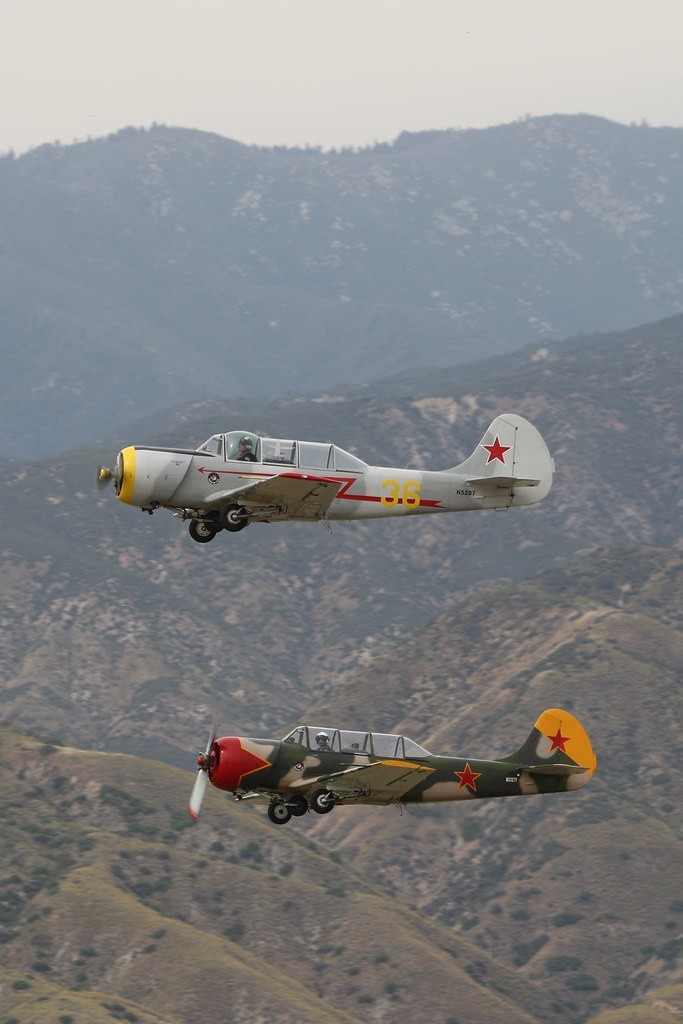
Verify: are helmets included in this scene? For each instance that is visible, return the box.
[315,732,328,745]
[241,436,253,449]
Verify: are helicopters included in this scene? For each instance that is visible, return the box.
[185,708,596,825]
[97,412,555,544]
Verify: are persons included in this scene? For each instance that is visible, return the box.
[236,437,257,462]
[315,732,335,752]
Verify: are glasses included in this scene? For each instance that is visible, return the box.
[316,735,327,740]
[239,441,246,446]
